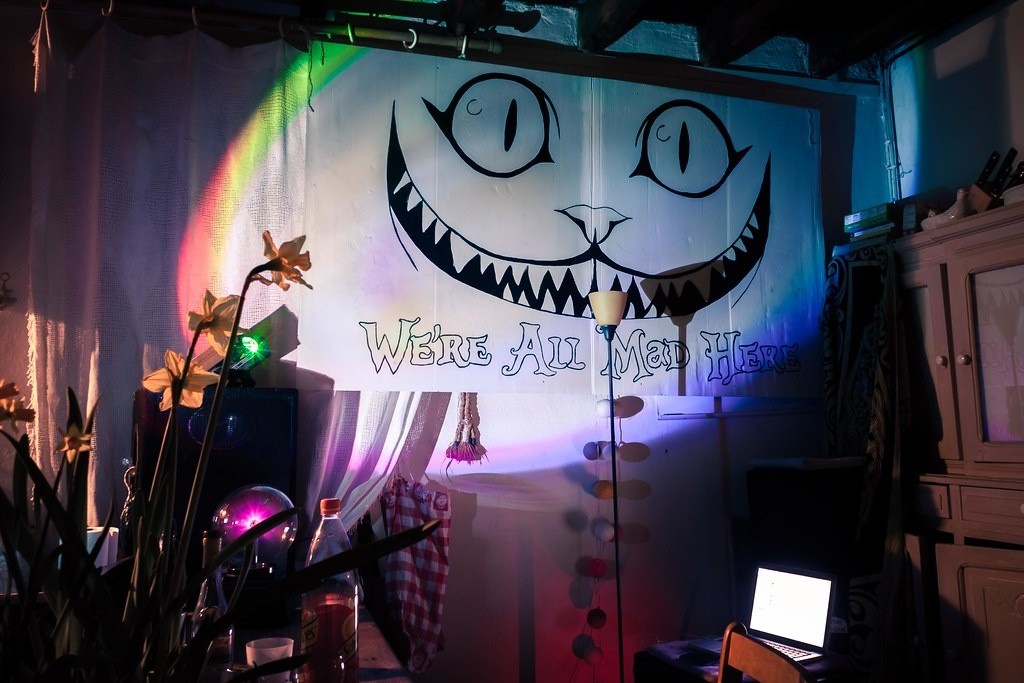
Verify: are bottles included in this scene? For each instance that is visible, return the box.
[300,497,359,683]
[191,530,234,673]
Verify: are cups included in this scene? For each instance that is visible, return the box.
[246,638,295,683]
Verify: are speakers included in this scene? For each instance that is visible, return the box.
[131,385,299,605]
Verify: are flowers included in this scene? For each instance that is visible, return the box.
[25,423,95,590]
[177,228,313,558]
[123,348,221,632]
[0,377,36,585]
[121,290,253,630]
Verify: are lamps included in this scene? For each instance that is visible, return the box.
[589,290,631,683]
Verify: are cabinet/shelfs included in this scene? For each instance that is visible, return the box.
[832,202,1024,683]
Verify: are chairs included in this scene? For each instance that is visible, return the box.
[717,621,818,683]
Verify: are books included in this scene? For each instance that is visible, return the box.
[832,222,902,257]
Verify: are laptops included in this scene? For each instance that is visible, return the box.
[686,561,839,666]
